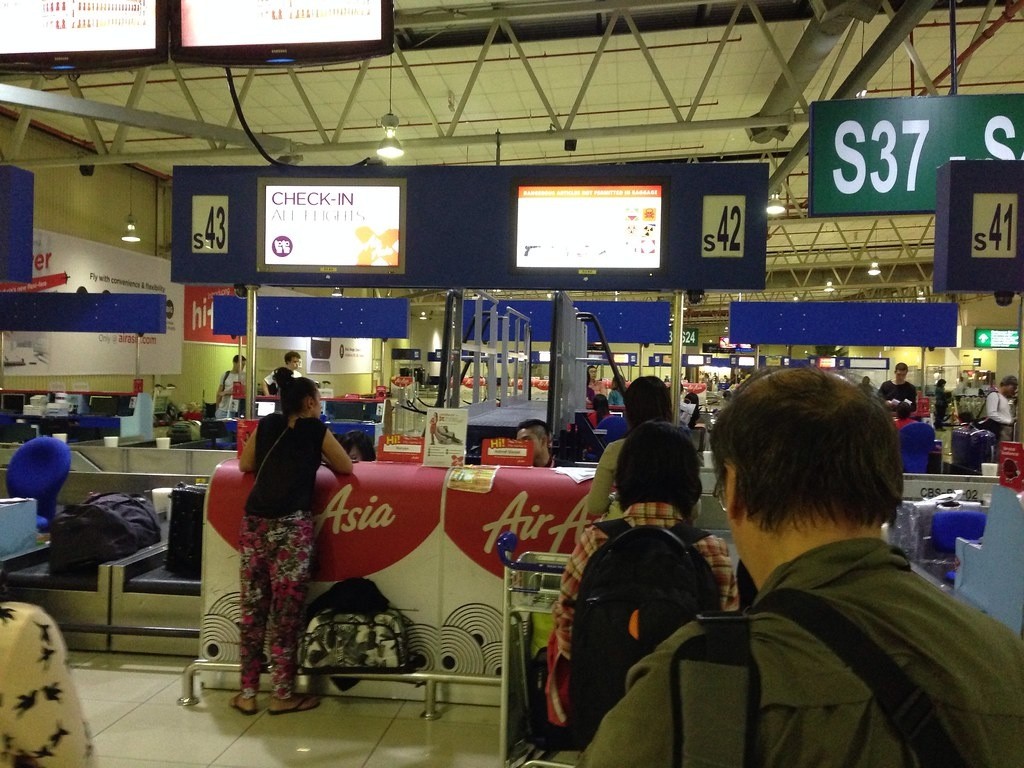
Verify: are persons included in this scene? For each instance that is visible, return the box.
[863,363,1018,445]
[227,367,357,715]
[339,429,374,461]
[215,355,246,440]
[556,365,758,750]
[578,363,1024,768]
[261,350,304,395]
[513,418,575,467]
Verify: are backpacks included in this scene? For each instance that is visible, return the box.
[567,517,720,733]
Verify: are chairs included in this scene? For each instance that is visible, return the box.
[931,511,988,581]
[897,423,936,477]
[586,414,628,461]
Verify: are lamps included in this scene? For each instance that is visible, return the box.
[766,139,785,214]
[823,249,835,292]
[867,222,882,275]
[121,166,141,243]
[376,53,405,158]
[154,383,176,394]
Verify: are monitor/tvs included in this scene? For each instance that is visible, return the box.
[2,394,24,412]
[256,402,275,416]
[509,178,671,277]
[256,177,406,274]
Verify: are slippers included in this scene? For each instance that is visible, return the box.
[265,694,321,715]
[228,692,259,715]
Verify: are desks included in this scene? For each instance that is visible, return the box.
[1,412,121,442]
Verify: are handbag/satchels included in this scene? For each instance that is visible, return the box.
[969,415,1000,441]
[46,488,161,580]
[297,590,409,673]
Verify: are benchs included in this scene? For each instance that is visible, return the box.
[5,537,202,657]
